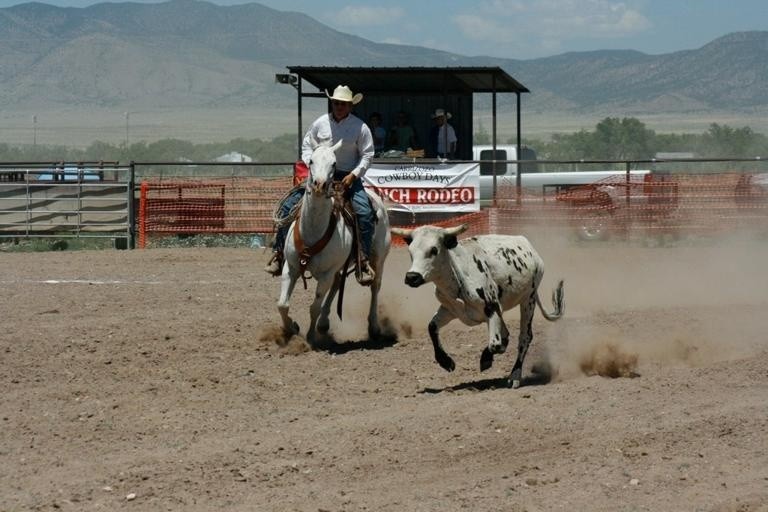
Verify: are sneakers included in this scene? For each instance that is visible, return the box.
[355,256,376,283]
[265,253,281,272]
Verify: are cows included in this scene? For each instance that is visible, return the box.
[389,223,565,389]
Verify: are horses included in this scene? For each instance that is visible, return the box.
[276,134,390,347]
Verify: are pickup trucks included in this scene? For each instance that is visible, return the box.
[473,144,680,243]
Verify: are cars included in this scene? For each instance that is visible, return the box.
[735,170,766,216]
[31,164,105,190]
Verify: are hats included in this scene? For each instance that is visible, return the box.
[430,109,452,119]
[325,84,363,105]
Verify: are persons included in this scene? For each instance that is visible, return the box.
[367,110,457,158]
[262,84,376,286]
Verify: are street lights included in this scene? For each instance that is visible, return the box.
[32,114,39,144]
[124,112,129,147]
